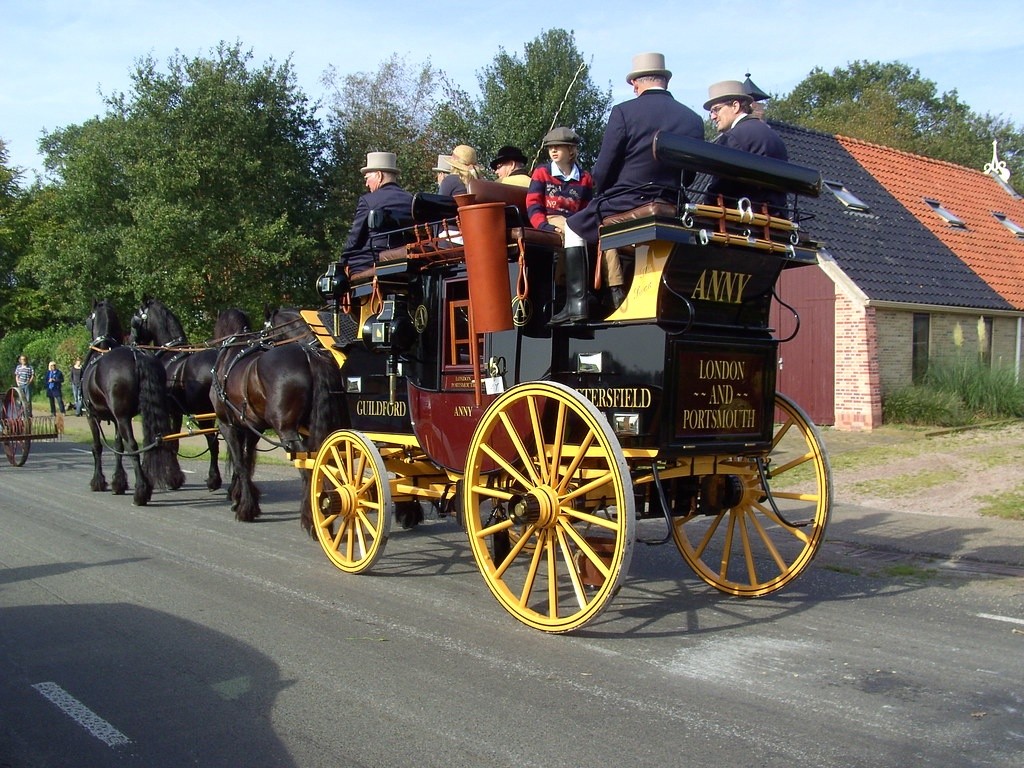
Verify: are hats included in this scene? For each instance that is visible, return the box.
[543,127,581,146]
[443,145,486,170]
[703,81,754,110]
[490,146,527,169]
[625,53,672,86]
[359,152,402,174]
[432,155,451,172]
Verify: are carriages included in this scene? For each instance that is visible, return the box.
[77,130,835,635]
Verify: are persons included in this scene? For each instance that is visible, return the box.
[45,361,66,417]
[428,144,480,258]
[340,151,414,275]
[525,126,627,313]
[547,52,705,323]
[70,356,84,417]
[702,80,790,220]
[489,145,532,188]
[431,154,453,185]
[14,355,35,418]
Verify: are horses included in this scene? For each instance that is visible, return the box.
[209,309,343,541]
[80,298,183,505]
[261,307,324,351]
[131,299,222,491]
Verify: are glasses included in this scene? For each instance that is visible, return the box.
[496,161,509,168]
[363,174,376,181]
[711,101,730,113]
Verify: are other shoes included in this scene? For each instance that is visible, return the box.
[601,286,625,308]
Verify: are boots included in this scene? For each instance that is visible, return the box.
[551,247,588,323]
